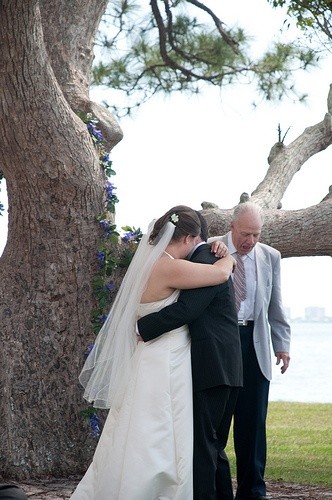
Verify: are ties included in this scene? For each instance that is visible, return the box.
[233,252,246,312]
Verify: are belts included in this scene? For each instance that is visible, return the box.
[238,319,254,326]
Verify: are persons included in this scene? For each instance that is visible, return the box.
[208,202,291,500]
[135,210,243,500]
[69,205,238,500]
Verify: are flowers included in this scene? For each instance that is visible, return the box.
[171,214,179,223]
